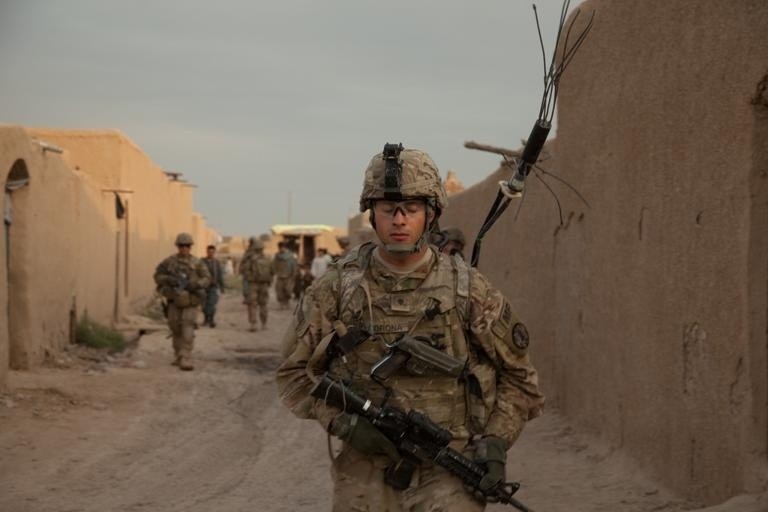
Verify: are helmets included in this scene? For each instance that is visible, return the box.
[441,228,466,246]
[174,232,194,245]
[358,149,447,213]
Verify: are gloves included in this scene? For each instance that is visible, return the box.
[186,282,201,291]
[462,434,508,503]
[167,276,182,287]
[343,416,401,464]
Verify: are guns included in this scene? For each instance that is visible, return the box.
[309,373,532,512]
[156,264,207,301]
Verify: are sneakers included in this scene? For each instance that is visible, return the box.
[179,349,194,370]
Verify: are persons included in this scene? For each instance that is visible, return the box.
[239,237,257,306]
[273,139,547,510]
[269,235,350,310]
[238,240,273,331]
[151,230,212,372]
[434,226,466,257]
[198,241,225,331]
